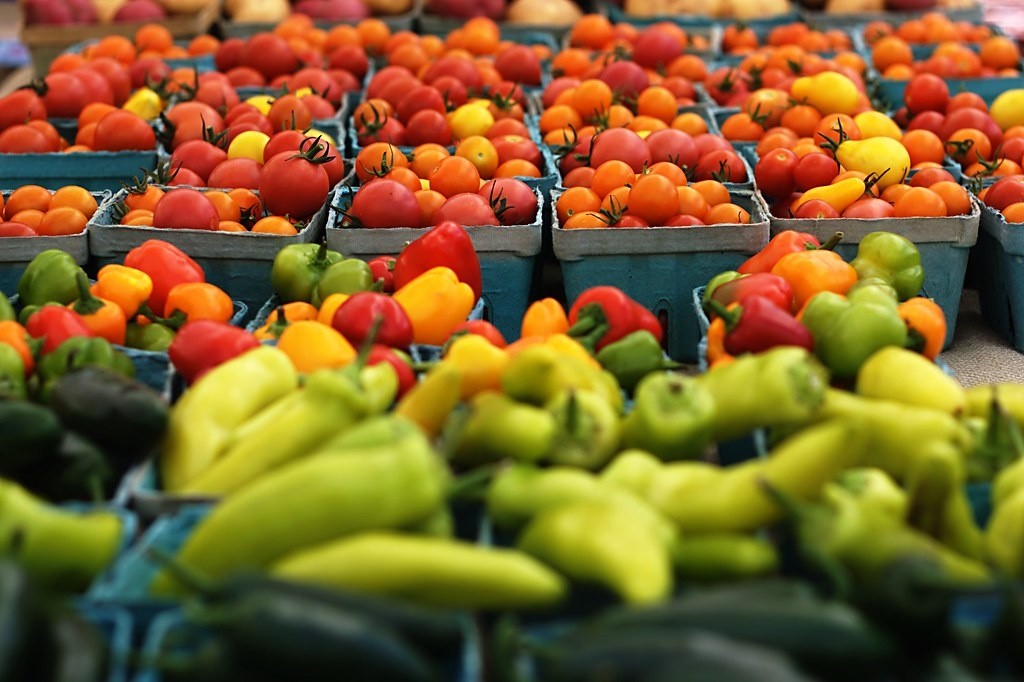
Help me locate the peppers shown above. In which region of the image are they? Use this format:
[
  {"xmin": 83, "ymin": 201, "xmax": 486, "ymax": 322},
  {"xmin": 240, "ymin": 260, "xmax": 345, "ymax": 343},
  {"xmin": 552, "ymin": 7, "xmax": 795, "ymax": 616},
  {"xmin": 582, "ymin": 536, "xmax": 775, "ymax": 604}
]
[{"xmin": 0, "ymin": 219, "xmax": 1023, "ymax": 682}]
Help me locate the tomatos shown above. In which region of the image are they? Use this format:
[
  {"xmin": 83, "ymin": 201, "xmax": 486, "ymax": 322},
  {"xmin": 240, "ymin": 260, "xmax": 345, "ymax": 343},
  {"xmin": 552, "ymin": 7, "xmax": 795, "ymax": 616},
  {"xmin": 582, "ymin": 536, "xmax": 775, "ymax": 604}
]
[{"xmin": 0, "ymin": 12, "xmax": 1023, "ymax": 237}]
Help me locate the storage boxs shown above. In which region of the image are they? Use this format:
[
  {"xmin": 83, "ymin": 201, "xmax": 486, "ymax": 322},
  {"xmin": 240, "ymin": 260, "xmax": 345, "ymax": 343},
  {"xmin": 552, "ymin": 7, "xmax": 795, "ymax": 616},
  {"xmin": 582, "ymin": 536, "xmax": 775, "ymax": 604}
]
[{"xmin": 0, "ymin": 0, "xmax": 1024, "ymax": 682}]
